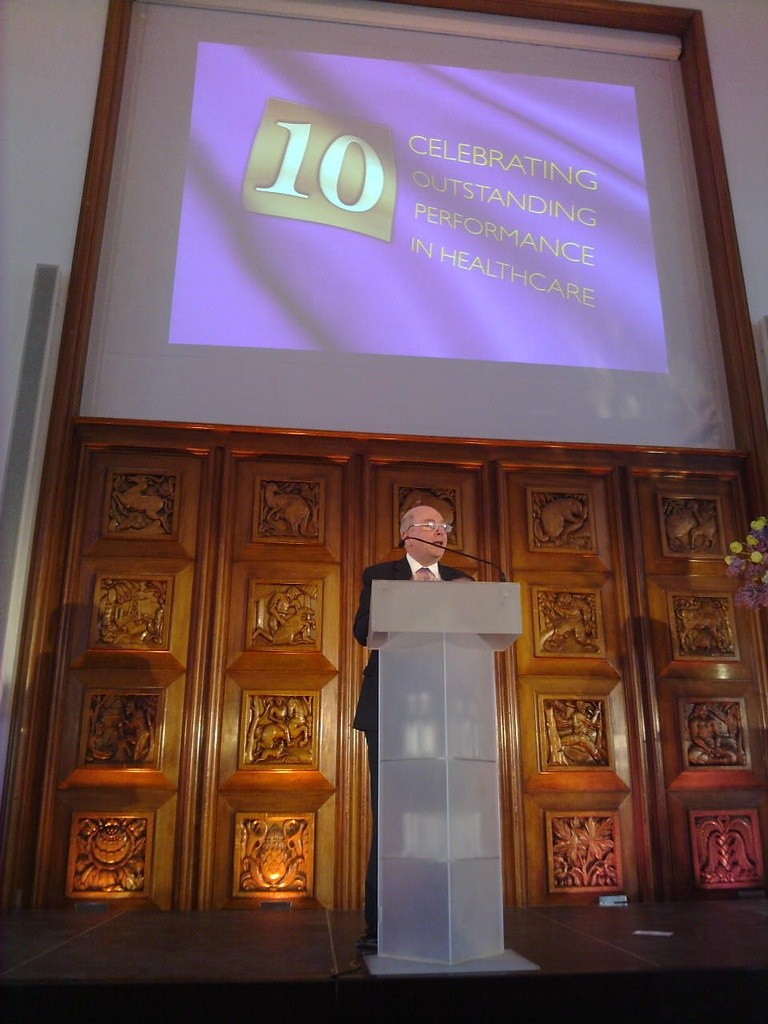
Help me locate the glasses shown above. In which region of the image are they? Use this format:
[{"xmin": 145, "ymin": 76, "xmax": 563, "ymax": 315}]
[{"xmin": 408, "ymin": 521, "xmax": 453, "ymax": 533}]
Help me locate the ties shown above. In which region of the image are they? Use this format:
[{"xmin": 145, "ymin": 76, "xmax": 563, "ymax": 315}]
[{"xmin": 416, "ymin": 568, "xmax": 430, "ymax": 581}]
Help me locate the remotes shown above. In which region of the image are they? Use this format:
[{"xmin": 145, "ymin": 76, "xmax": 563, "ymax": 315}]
[{"xmin": 398, "ymin": 536, "xmax": 507, "ymax": 582}]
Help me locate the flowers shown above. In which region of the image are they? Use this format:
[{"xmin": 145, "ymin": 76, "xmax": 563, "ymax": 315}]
[{"xmin": 725, "ymin": 516, "xmax": 767, "ymax": 610}]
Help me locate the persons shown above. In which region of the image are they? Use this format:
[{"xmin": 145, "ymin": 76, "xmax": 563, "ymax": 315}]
[{"xmin": 343, "ymin": 506, "xmax": 477, "ymax": 951}]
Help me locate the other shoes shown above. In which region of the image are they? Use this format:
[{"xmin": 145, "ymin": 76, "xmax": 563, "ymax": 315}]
[{"xmin": 356, "ymin": 924, "xmax": 377, "ymax": 949}]
